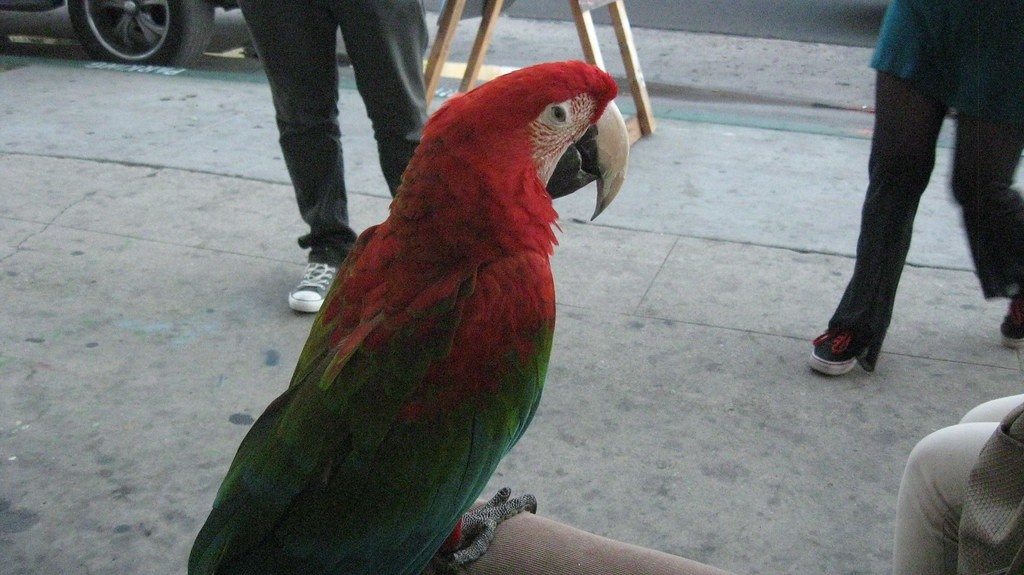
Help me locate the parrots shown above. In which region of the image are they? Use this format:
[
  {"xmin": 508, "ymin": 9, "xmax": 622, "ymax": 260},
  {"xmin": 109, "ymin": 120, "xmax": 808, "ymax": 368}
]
[{"xmin": 187, "ymin": 60, "xmax": 630, "ymax": 575}]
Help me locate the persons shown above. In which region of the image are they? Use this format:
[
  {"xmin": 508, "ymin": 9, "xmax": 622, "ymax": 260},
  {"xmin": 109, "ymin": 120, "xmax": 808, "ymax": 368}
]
[
  {"xmin": 809, "ymin": 0, "xmax": 1024, "ymax": 375},
  {"xmin": 237, "ymin": 1, "xmax": 430, "ymax": 313},
  {"xmin": 891, "ymin": 392, "xmax": 1024, "ymax": 574}
]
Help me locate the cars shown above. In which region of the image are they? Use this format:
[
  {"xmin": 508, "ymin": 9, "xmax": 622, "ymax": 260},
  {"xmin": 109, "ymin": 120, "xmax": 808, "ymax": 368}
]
[{"xmin": 0, "ymin": 1, "xmax": 242, "ymax": 69}]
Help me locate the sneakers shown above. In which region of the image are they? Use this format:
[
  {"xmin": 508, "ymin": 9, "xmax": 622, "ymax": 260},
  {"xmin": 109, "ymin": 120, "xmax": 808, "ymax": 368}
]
[
  {"xmin": 1000, "ymin": 295, "xmax": 1024, "ymax": 347},
  {"xmin": 811, "ymin": 329, "xmax": 867, "ymax": 377},
  {"xmin": 288, "ymin": 261, "xmax": 338, "ymax": 313}
]
